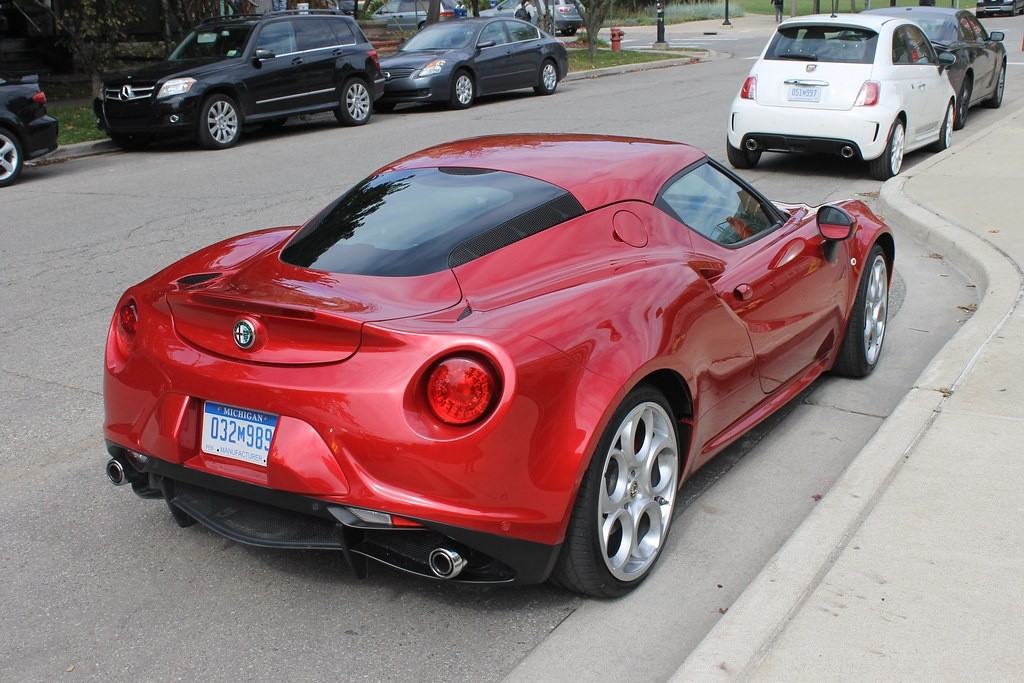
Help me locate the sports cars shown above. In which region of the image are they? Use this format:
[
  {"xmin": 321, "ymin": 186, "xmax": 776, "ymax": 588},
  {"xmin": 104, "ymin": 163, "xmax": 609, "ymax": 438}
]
[{"xmin": 101, "ymin": 133, "xmax": 898, "ymax": 598}]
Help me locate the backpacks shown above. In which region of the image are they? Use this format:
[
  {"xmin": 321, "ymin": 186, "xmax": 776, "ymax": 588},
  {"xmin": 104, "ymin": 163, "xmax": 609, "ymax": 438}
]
[{"xmin": 515, "ymin": 3, "xmax": 529, "ymax": 20}]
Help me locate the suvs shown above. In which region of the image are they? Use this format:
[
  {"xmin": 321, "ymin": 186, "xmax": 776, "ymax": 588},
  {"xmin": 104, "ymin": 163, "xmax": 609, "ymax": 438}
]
[{"xmin": 92, "ymin": 9, "xmax": 386, "ymax": 150}]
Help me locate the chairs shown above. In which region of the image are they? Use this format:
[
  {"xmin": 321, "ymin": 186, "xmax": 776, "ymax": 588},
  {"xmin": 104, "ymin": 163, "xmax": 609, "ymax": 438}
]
[
  {"xmin": 206, "ymin": 35, "xmax": 238, "ymax": 58},
  {"xmin": 863, "ymin": 29, "xmax": 904, "ymax": 63},
  {"xmin": 451, "ymin": 31, "xmax": 466, "ymax": 48},
  {"xmin": 802, "ymin": 31, "xmax": 826, "ymax": 50}
]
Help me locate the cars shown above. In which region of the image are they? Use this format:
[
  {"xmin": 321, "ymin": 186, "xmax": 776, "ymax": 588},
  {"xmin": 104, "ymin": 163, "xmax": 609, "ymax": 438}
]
[
  {"xmin": 838, "ymin": 6, "xmax": 1007, "ymax": 133},
  {"xmin": 975, "ymin": 0, "xmax": 1024, "ymax": 16},
  {"xmin": 479, "ymin": 0, "xmax": 586, "ymax": 35},
  {"xmin": 728, "ymin": 13, "xmax": 957, "ymax": 182},
  {"xmin": 373, "ymin": 16, "xmax": 570, "ymax": 111},
  {"xmin": 369, "ymin": 0, "xmax": 467, "ymax": 31},
  {"xmin": 1, "ymin": 74, "xmax": 61, "ymax": 187}
]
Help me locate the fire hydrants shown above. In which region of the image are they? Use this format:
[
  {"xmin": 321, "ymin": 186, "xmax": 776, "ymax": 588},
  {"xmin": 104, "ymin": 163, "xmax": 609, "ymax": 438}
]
[{"xmin": 609, "ymin": 26, "xmax": 625, "ymax": 51}]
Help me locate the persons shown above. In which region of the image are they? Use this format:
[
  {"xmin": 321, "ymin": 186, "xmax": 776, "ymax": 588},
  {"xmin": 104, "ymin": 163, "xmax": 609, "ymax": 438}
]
[
  {"xmin": 454, "ymin": 0, "xmax": 467, "ymax": 19},
  {"xmin": 514, "ymin": 0, "xmax": 535, "ymax": 23}
]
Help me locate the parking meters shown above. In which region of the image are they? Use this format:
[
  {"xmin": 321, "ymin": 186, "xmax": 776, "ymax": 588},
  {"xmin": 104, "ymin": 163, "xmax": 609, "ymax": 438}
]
[{"xmin": 454, "ymin": 4, "xmax": 467, "ymax": 18}]
